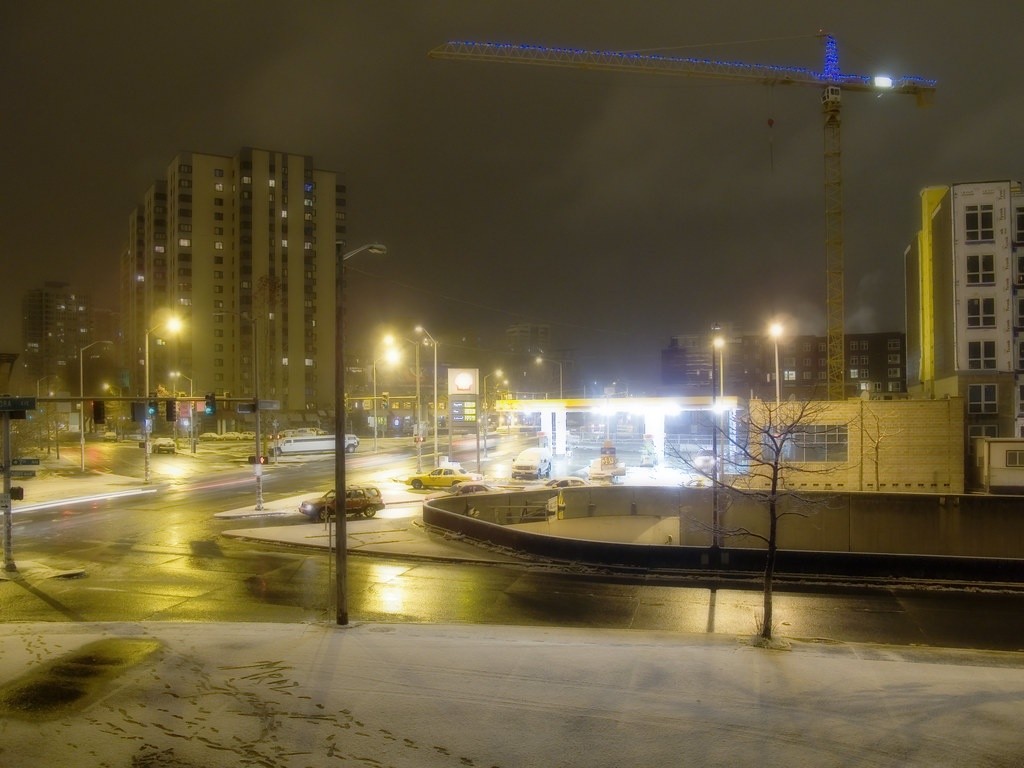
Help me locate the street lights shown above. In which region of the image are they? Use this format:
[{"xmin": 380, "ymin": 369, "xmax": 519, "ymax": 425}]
[
  {"xmin": 414, "ymin": 326, "xmax": 439, "ymax": 470},
  {"xmin": 81, "ymin": 339, "xmax": 116, "ymax": 473},
  {"xmin": 767, "ymin": 322, "xmax": 782, "ymax": 434},
  {"xmin": 711, "ymin": 337, "xmax": 725, "ymax": 486},
  {"xmin": 583, "ymin": 381, "xmax": 596, "ymax": 399},
  {"xmin": 372, "ymin": 353, "xmax": 399, "ymax": 453},
  {"xmin": 36, "ymin": 374, "xmax": 60, "ymax": 449},
  {"xmin": 612, "ymin": 381, "xmax": 629, "ymax": 398},
  {"xmin": 144, "ymin": 316, "xmax": 182, "ymax": 484},
  {"xmin": 482, "ymin": 369, "xmax": 502, "ymax": 458},
  {"xmin": 383, "ymin": 335, "xmax": 423, "ymax": 471},
  {"xmin": 335, "ymin": 237, "xmax": 388, "ymax": 626},
  {"xmin": 212, "ymin": 310, "xmax": 264, "ymax": 513},
  {"xmin": 175, "ymin": 372, "xmax": 194, "ymax": 452},
  {"xmin": 535, "ymin": 356, "xmax": 562, "ymax": 400},
  {"xmin": 503, "ymin": 380, "xmax": 509, "ymax": 401}
]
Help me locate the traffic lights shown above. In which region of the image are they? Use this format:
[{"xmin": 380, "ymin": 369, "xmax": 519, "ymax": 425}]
[
  {"xmin": 148, "ymin": 400, "xmax": 158, "ymax": 416},
  {"xmin": 204, "ymin": 394, "xmax": 216, "ymax": 415},
  {"xmin": 382, "ymin": 392, "xmax": 390, "ymax": 409}
]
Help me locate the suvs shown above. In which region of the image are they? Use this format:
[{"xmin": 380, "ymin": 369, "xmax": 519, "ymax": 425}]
[
  {"xmin": 695, "ymin": 448, "xmax": 721, "ymax": 475},
  {"xmin": 512, "ymin": 447, "xmax": 553, "ymax": 479},
  {"xmin": 298, "ymin": 483, "xmax": 386, "ymax": 522}
]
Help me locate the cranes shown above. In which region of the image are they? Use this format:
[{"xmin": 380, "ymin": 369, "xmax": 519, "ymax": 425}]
[{"xmin": 424, "ymin": 30, "xmax": 938, "ymax": 404}]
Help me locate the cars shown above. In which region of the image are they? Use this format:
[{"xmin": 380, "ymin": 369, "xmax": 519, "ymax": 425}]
[
  {"xmin": 523, "ymin": 476, "xmax": 601, "ymax": 492},
  {"xmin": 405, "ymin": 466, "xmax": 484, "ymax": 490},
  {"xmin": 153, "ymin": 438, "xmax": 176, "ymax": 455},
  {"xmin": 268, "ymin": 434, "xmax": 359, "ymax": 458},
  {"xmin": 424, "ymin": 481, "xmax": 505, "ymax": 503}
]
